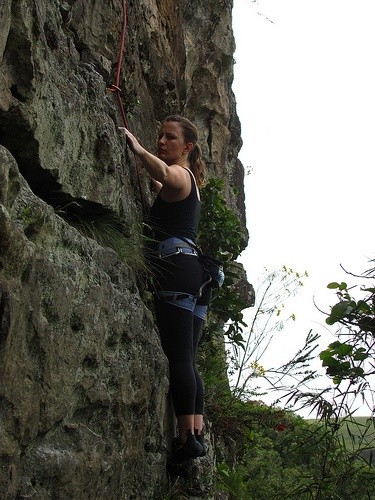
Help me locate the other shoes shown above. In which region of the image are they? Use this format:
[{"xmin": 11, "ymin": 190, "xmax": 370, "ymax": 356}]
[{"xmin": 173, "ymin": 428, "xmax": 206, "ymax": 460}]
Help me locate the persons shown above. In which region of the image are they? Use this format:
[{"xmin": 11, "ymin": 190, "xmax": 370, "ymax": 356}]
[{"xmin": 117, "ymin": 114, "xmax": 213, "ymax": 464}]
